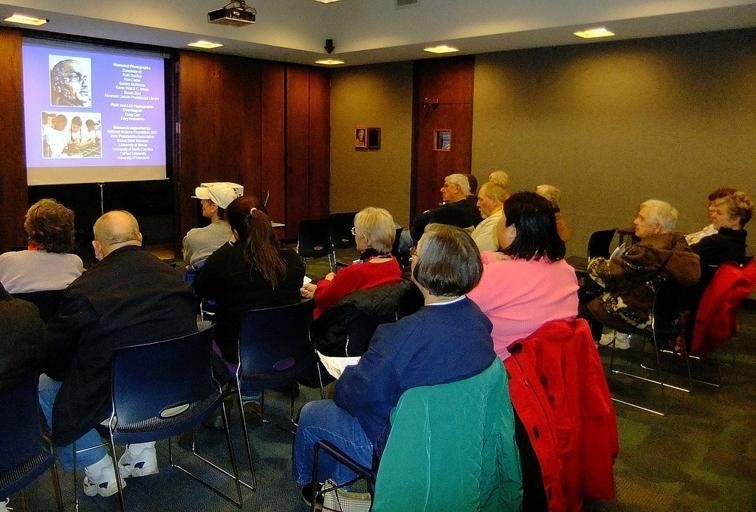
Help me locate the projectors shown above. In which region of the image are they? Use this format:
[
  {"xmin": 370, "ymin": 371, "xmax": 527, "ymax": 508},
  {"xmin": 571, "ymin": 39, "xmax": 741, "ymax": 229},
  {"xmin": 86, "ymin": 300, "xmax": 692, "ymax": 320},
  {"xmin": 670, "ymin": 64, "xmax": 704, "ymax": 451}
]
[{"xmin": 207, "ymin": 9, "xmax": 255, "ymax": 28}]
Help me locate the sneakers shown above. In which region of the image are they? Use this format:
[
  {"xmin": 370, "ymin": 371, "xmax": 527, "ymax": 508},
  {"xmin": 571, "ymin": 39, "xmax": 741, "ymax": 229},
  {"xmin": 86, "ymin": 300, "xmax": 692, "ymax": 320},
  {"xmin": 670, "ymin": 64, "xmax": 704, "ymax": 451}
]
[
  {"xmin": 83, "ymin": 456, "xmax": 125, "ymax": 497},
  {"xmin": 598, "ymin": 331, "xmax": 629, "ymax": 350},
  {"xmin": 117, "ymin": 449, "xmax": 159, "ymax": 480},
  {"xmin": 301, "ymin": 483, "xmax": 323, "ymax": 510}
]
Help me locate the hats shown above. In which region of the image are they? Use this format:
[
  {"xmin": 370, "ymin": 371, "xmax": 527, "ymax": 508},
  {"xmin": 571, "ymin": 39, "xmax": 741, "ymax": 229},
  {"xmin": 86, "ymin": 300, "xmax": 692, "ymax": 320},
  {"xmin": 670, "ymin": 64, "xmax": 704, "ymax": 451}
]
[{"xmin": 194, "ymin": 185, "xmax": 237, "ymax": 209}]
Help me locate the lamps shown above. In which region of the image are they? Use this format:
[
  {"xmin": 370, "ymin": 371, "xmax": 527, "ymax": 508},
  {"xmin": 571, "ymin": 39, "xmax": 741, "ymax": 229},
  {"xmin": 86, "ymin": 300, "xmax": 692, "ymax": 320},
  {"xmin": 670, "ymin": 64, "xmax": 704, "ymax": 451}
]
[{"xmin": 207, "ymin": 0, "xmax": 257, "ymax": 26}]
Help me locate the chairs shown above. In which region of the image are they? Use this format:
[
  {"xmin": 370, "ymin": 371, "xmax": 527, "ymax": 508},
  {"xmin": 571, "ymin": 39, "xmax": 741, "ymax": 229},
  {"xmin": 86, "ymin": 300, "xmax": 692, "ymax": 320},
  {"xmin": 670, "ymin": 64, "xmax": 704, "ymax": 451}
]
[
  {"xmin": 319, "ymin": 272, "xmax": 427, "ymax": 353},
  {"xmin": 606, "ymin": 285, "xmax": 697, "ymax": 417},
  {"xmin": 560, "ymin": 226, "xmax": 615, "ymax": 279},
  {"xmin": 313, "ymin": 367, "xmax": 505, "ymax": 512},
  {"xmin": 506, "ymin": 313, "xmax": 620, "ymax": 509},
  {"xmin": 298, "ymin": 212, "xmax": 401, "ymax": 263},
  {"xmin": 0, "ymin": 358, "xmax": 65, "ymax": 512},
  {"xmin": 74, "ymin": 324, "xmax": 244, "ymax": 511},
  {"xmin": 193, "ymin": 298, "xmax": 325, "ymax": 493},
  {"xmin": 702, "ymin": 255, "xmax": 754, "ymax": 392}
]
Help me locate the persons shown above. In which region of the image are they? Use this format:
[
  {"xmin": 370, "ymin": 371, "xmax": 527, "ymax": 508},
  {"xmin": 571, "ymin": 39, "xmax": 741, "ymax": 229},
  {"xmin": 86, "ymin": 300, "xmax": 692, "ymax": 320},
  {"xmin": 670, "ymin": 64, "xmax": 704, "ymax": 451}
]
[
  {"xmin": 42, "ymin": 111, "xmax": 102, "ymax": 159},
  {"xmin": 470, "ymin": 170, "xmax": 511, "ymax": 249},
  {"xmin": 182, "ymin": 183, "xmax": 237, "ymax": 270},
  {"xmin": 0, "ymin": 199, "xmax": 83, "ymax": 292},
  {"xmin": 398, "ymin": 173, "xmax": 484, "ymax": 252},
  {"xmin": 300, "ymin": 206, "xmax": 401, "ymax": 319},
  {"xmin": 51, "ymin": 58, "xmax": 91, "ymax": 106},
  {"xmin": 684, "ymin": 186, "xmax": 753, "ymax": 268},
  {"xmin": 537, "ymin": 185, "xmax": 570, "ymax": 243},
  {"xmin": 356, "ymin": 129, "xmax": 365, "ymax": 146},
  {"xmin": 41, "ymin": 211, "xmax": 184, "ymax": 497},
  {"xmin": 293, "ymin": 223, "xmax": 497, "ymax": 510},
  {"xmin": 466, "ymin": 191, "xmax": 580, "ymax": 358},
  {"xmin": 585, "ymin": 200, "xmax": 700, "ymax": 350},
  {"xmin": 201, "ymin": 196, "xmax": 306, "ymax": 421}
]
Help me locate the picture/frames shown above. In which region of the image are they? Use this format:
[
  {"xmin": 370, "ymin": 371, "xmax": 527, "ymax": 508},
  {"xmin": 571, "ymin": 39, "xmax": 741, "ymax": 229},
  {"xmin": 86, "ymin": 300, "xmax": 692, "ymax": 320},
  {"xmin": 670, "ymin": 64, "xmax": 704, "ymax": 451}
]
[
  {"xmin": 354, "ymin": 128, "xmax": 381, "ymax": 150},
  {"xmin": 433, "ymin": 129, "xmax": 452, "ymax": 152}
]
